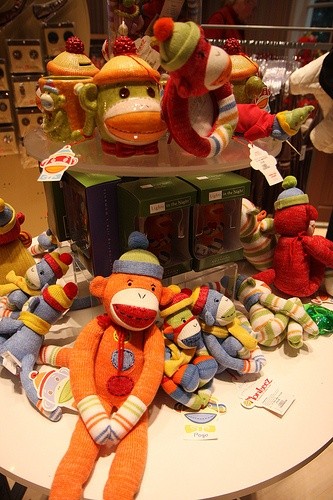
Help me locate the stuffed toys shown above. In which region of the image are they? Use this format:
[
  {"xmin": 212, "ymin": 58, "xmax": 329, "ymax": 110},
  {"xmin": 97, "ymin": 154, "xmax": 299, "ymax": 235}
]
[
  {"xmin": 252, "ymin": 175, "xmax": 333, "ymax": 297},
  {"xmin": 49, "ymin": 231, "xmax": 173, "ymax": 500},
  {"xmin": 197, "ymin": 203, "xmax": 225, "ymax": 255},
  {"xmin": 221, "ymin": 274, "xmax": 319, "ymax": 349},
  {"xmin": 0, "ymin": 197, "xmax": 78, "ymax": 422},
  {"xmin": 181, "ymin": 286, "xmax": 266, "ymax": 376},
  {"xmin": 239, "ymin": 198, "xmax": 277, "ymax": 272},
  {"xmin": 154, "ymin": 18, "xmax": 239, "ymax": 160},
  {"xmin": 145, "ymin": 214, "xmax": 176, "ymax": 262},
  {"xmin": 157, "ymin": 285, "xmax": 217, "ymax": 410},
  {"xmin": 234, "ymin": 105, "xmax": 315, "ymax": 142}
]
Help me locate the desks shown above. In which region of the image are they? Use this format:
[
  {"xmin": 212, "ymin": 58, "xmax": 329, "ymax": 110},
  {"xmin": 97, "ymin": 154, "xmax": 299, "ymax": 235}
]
[
  {"xmin": 24, "ymin": 125, "xmax": 282, "ymax": 179},
  {"xmin": 0, "ymin": 295, "xmax": 333, "ymax": 500}
]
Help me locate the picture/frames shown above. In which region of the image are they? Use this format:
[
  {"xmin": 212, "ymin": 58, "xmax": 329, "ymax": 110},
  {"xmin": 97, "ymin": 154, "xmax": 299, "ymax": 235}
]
[{"xmin": 197, "ymin": 0, "xmax": 297, "ymax": 61}]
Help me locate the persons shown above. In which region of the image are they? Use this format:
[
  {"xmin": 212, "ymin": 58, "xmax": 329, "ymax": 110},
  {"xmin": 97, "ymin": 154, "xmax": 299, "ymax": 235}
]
[{"xmin": 204, "ymin": 0, "xmax": 257, "ymax": 55}]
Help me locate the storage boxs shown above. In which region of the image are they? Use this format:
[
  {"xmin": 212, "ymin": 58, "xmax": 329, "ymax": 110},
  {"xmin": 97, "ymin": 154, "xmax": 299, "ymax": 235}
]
[
  {"xmin": 61, "ymin": 170, "xmax": 122, "ymax": 279},
  {"xmin": 167, "ymin": 262, "xmax": 238, "ymax": 303},
  {"xmin": 10, "ymin": 74, "xmax": 43, "ymax": 110},
  {"xmin": 14, "ymin": 108, "xmax": 44, "ymax": 141},
  {"xmin": 0, "ymin": 124, "xmax": 19, "ymax": 157},
  {"xmin": 0, "ymin": 57, "xmax": 10, "ymax": 94},
  {"xmin": 5, "ymin": 39, "xmax": 46, "ymax": 75},
  {"xmin": 42, "ymin": 21, "xmax": 76, "ymax": 59},
  {"xmin": 17, "ymin": 139, "xmax": 39, "ymax": 169},
  {"xmin": 0, "ymin": 94, "xmax": 15, "ymax": 126},
  {"xmin": 117, "ymin": 176, "xmax": 197, "ymax": 279},
  {"xmin": 55, "ymin": 241, "xmax": 103, "ymax": 314},
  {"xmin": 38, "ymin": 160, "xmax": 70, "ymax": 242},
  {"xmin": 175, "ymin": 171, "xmax": 252, "ymax": 273}
]
